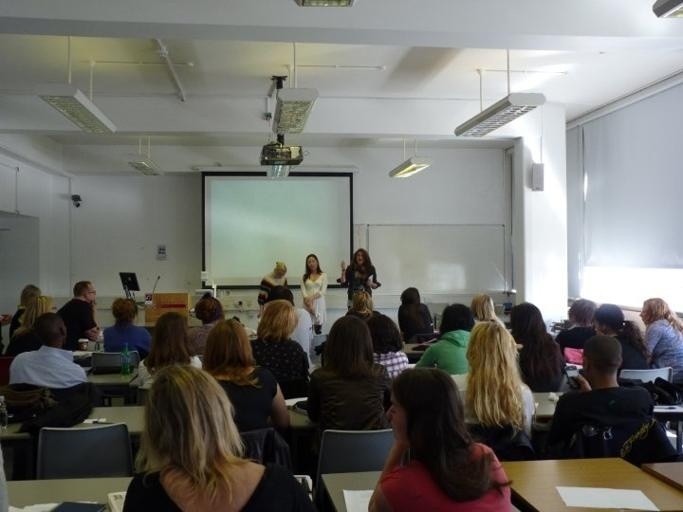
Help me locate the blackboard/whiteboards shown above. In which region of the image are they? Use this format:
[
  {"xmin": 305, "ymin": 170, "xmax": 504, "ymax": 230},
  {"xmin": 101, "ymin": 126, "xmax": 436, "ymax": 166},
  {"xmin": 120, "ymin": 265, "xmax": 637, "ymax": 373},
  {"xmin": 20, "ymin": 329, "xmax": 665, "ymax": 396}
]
[{"xmin": 367, "ymin": 223, "xmax": 507, "ymax": 295}]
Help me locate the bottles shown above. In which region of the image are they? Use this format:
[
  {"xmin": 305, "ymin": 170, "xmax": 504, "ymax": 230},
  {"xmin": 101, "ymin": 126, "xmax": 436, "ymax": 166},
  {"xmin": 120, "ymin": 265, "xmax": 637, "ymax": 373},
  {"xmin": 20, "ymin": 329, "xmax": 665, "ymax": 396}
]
[
  {"xmin": 0, "ymin": 396, "xmax": 8, "ymax": 433},
  {"xmin": 118, "ymin": 342, "xmax": 131, "ymax": 381}
]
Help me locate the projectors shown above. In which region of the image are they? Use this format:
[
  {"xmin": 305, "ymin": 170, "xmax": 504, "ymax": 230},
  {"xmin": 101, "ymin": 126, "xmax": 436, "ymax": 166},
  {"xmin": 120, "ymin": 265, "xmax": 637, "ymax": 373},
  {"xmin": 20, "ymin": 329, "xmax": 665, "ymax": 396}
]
[{"xmin": 260, "ymin": 144, "xmax": 303, "ymax": 165}]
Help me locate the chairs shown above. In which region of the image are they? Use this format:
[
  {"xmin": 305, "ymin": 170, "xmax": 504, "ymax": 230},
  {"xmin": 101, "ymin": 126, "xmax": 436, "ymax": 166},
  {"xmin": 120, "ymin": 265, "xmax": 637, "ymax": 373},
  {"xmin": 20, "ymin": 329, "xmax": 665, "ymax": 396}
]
[{"xmin": 1, "ymin": 343, "xmax": 683, "ymax": 512}]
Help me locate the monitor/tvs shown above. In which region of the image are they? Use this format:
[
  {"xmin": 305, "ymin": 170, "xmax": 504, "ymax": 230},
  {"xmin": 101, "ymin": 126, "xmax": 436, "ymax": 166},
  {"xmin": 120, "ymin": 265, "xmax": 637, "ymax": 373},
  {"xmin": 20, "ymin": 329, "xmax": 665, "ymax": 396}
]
[{"xmin": 119, "ymin": 271, "xmax": 140, "ymax": 291}]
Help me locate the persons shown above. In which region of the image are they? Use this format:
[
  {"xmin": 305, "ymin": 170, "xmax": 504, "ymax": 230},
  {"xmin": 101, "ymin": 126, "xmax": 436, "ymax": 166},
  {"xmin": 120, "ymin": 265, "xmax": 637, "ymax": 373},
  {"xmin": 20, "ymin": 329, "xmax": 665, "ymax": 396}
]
[
  {"xmin": 368, "ymin": 368, "xmax": 513, "ymax": 511},
  {"xmin": 0, "ymin": 282, "xmax": 683, "ymax": 460},
  {"xmin": 122, "ymin": 360, "xmax": 315, "ymax": 511},
  {"xmin": 257, "ymin": 261, "xmax": 289, "ymax": 318},
  {"xmin": 340, "ymin": 248, "xmax": 381, "ymax": 309},
  {"xmin": 299, "ymin": 253, "xmax": 328, "ymax": 336}
]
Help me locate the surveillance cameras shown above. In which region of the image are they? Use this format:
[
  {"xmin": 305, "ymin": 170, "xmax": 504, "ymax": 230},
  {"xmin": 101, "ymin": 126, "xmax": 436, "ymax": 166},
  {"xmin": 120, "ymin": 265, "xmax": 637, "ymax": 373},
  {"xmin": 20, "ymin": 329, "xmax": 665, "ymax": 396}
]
[{"xmin": 70, "ymin": 193, "xmax": 82, "ymax": 207}]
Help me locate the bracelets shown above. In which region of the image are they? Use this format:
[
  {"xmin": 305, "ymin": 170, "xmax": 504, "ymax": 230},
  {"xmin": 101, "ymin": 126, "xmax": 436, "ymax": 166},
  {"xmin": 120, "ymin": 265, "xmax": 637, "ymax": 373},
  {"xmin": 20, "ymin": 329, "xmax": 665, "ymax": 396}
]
[{"xmin": 342, "ymin": 268, "xmax": 346, "ymax": 271}]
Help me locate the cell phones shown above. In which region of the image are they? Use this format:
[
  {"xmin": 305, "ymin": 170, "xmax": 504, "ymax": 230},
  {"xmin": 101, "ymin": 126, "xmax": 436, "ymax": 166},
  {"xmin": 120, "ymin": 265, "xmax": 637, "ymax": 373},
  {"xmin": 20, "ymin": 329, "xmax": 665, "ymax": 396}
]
[{"xmin": 564, "ymin": 365, "xmax": 581, "ymax": 389}]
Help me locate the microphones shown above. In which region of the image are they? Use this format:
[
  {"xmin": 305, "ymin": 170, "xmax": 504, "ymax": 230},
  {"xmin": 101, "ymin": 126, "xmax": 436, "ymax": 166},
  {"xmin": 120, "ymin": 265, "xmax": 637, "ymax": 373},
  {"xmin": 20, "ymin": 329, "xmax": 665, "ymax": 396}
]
[{"xmin": 151, "ymin": 275, "xmax": 161, "ymax": 293}]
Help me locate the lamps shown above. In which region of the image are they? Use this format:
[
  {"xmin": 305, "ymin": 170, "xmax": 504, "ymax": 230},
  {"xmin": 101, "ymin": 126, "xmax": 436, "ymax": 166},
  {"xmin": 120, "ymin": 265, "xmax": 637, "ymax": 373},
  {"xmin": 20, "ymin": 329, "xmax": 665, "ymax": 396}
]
[
  {"xmin": 653, "ymin": 1, "xmax": 683, "ymax": 20},
  {"xmin": 35, "ymin": 36, "xmax": 116, "ymax": 135},
  {"xmin": 128, "ymin": 137, "xmax": 165, "ymax": 176},
  {"xmin": 390, "ymin": 139, "xmax": 432, "ymax": 178},
  {"xmin": 273, "ymin": 42, "xmax": 318, "ymax": 135},
  {"xmin": 454, "ymin": 48, "xmax": 545, "ymax": 137}
]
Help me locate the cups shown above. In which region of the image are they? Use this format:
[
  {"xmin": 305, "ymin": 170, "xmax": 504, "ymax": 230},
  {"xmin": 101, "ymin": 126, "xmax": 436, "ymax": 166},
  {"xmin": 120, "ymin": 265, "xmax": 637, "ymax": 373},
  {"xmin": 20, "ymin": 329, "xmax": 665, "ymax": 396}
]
[{"xmin": 78, "ymin": 338, "xmax": 89, "ymax": 351}]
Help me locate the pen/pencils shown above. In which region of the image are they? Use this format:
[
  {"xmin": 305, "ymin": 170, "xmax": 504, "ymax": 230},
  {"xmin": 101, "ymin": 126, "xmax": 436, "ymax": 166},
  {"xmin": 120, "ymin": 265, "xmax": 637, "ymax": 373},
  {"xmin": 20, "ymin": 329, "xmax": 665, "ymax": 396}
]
[
  {"xmin": 93, "ymin": 421, "xmax": 114, "ymax": 425},
  {"xmin": 654, "ymin": 406, "xmax": 676, "ymax": 409},
  {"xmin": 129, "ymin": 374, "xmax": 139, "ymax": 383}
]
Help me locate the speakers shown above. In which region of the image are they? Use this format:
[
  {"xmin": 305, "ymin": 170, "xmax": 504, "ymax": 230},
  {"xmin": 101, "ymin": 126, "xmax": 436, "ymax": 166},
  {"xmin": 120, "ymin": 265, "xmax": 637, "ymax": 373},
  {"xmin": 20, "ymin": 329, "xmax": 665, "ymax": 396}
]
[{"xmin": 531, "ymin": 164, "xmax": 544, "ymax": 190}]
